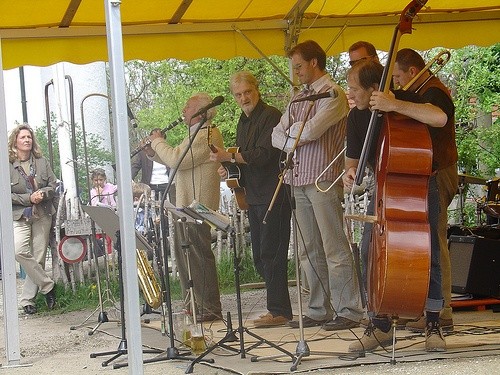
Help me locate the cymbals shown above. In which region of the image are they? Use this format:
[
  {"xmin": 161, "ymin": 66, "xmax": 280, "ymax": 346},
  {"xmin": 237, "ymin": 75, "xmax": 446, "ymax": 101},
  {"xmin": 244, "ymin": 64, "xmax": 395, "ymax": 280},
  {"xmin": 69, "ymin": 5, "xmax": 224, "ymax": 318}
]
[
  {"xmin": 344, "ymin": 215, "xmax": 378, "ymax": 223},
  {"xmin": 458, "ymin": 174, "xmax": 489, "ymax": 185},
  {"xmin": 113, "ymin": 183, "xmax": 151, "ymax": 203}
]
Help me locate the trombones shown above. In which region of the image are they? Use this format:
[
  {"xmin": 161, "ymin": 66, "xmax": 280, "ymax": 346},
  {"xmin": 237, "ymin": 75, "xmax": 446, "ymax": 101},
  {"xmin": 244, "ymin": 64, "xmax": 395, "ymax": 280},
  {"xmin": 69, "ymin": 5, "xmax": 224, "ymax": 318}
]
[{"xmin": 314, "ymin": 50, "xmax": 452, "ymax": 193}]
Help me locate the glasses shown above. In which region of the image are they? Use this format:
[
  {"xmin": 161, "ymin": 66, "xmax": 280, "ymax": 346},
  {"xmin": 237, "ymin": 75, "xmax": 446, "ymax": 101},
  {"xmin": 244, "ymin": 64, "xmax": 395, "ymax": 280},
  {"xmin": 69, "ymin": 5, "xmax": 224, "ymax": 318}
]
[
  {"xmin": 349, "ymin": 54, "xmax": 373, "ymax": 67},
  {"xmin": 292, "ymin": 60, "xmax": 306, "ymax": 71}
]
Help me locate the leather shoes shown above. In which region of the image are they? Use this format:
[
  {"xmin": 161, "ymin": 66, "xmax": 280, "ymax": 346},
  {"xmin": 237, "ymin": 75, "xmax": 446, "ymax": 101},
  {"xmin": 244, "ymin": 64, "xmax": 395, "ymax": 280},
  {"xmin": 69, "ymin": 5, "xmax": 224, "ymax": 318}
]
[
  {"xmin": 23, "ymin": 305, "xmax": 37, "ymax": 314},
  {"xmin": 44, "ymin": 281, "xmax": 57, "ymax": 310}
]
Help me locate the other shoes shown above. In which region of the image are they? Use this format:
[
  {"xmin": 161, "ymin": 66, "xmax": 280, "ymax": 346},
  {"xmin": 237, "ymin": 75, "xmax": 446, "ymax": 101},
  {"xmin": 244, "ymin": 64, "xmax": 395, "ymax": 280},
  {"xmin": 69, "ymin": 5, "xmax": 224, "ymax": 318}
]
[
  {"xmin": 288, "ymin": 315, "xmax": 333, "ymax": 328},
  {"xmin": 191, "ymin": 310, "xmax": 224, "ymax": 323},
  {"xmin": 178, "ymin": 305, "xmax": 200, "ymax": 316},
  {"xmin": 321, "ymin": 316, "xmax": 361, "ymax": 331}
]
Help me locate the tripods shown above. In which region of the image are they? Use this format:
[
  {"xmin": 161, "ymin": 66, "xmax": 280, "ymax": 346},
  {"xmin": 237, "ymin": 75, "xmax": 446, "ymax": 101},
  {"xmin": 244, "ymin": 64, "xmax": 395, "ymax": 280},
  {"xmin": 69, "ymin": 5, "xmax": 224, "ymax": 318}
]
[{"xmin": 70, "ymin": 94, "xmax": 365, "ymax": 373}]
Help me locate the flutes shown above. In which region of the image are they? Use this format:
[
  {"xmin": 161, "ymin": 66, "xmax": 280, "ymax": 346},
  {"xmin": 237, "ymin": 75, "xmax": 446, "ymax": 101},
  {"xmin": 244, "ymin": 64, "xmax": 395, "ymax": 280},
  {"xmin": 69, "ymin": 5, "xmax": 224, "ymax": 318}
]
[{"xmin": 111, "ymin": 116, "xmax": 185, "ymax": 171}]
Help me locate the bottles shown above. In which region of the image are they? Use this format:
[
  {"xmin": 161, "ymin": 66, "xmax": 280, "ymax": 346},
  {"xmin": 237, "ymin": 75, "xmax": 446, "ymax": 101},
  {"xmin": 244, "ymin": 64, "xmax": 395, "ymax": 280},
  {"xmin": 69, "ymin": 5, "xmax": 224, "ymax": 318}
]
[{"xmin": 182, "ymin": 308, "xmax": 194, "ymax": 350}]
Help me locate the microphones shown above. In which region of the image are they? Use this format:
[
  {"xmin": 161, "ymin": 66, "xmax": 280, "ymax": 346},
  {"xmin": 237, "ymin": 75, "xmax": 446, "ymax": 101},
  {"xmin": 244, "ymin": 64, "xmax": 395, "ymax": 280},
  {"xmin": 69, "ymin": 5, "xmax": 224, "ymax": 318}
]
[
  {"xmin": 293, "ymin": 89, "xmax": 338, "ymax": 103},
  {"xmin": 191, "ymin": 96, "xmax": 224, "ymax": 118},
  {"xmin": 126, "ymin": 104, "xmax": 138, "ymax": 128}
]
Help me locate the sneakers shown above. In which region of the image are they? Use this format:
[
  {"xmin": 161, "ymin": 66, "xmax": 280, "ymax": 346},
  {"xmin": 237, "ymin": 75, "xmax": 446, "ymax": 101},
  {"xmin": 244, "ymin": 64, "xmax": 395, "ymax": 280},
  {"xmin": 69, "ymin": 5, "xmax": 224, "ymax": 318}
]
[
  {"xmin": 422, "ymin": 321, "xmax": 447, "ymax": 351},
  {"xmin": 407, "ymin": 313, "xmax": 454, "ymax": 332},
  {"xmin": 348, "ymin": 323, "xmax": 397, "ymax": 351},
  {"xmin": 254, "ymin": 312, "xmax": 292, "ymax": 325},
  {"xmin": 392, "ymin": 312, "xmax": 424, "ymax": 330}
]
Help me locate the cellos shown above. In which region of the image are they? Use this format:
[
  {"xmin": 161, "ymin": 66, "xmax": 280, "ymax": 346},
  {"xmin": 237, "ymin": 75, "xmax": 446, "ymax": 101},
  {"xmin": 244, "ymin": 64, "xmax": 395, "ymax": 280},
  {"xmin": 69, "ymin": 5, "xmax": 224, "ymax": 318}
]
[{"xmin": 343, "ymin": 0, "xmax": 435, "ymax": 366}]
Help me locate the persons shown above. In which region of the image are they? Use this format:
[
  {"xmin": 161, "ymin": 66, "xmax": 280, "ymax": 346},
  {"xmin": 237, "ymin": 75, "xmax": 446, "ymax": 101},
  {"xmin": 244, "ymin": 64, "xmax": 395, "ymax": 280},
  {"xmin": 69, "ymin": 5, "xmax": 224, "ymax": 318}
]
[
  {"xmin": 270, "ymin": 41, "xmax": 363, "ymax": 330},
  {"xmin": 344, "ymin": 41, "xmax": 460, "ymax": 355},
  {"xmin": 217, "ymin": 73, "xmax": 296, "ymax": 327},
  {"xmin": 90, "ymin": 168, "xmax": 117, "ymax": 212},
  {"xmin": 132, "ymin": 182, "xmax": 161, "ymax": 237},
  {"xmin": 141, "ymin": 93, "xmax": 227, "ymax": 322},
  {"xmin": 8, "ymin": 123, "xmax": 58, "ymax": 313}
]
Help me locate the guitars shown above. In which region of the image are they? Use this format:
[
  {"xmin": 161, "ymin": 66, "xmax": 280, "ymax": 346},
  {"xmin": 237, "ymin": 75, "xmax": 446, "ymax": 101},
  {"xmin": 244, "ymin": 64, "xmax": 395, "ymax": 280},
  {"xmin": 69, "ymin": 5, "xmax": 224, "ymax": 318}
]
[{"xmin": 207, "ymin": 126, "xmax": 249, "ymax": 210}]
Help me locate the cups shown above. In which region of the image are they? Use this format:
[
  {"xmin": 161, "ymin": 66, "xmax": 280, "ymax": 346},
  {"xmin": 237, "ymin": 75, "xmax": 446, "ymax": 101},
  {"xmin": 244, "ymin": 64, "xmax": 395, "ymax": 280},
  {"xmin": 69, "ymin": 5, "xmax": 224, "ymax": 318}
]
[{"xmin": 189, "ymin": 324, "xmax": 206, "ymax": 355}]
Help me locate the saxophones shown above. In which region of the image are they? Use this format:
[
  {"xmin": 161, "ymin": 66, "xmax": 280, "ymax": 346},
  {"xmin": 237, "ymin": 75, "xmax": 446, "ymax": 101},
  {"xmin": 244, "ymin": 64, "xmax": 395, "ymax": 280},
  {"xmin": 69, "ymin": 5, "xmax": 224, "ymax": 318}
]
[{"xmin": 137, "ymin": 249, "xmax": 162, "ymax": 309}]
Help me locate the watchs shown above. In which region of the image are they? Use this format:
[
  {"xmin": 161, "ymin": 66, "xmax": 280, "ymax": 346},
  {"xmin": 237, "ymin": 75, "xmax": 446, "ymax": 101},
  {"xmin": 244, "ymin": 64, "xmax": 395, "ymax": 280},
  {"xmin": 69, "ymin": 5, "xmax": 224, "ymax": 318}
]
[{"xmin": 231, "ymin": 153, "xmax": 236, "ymax": 164}]
